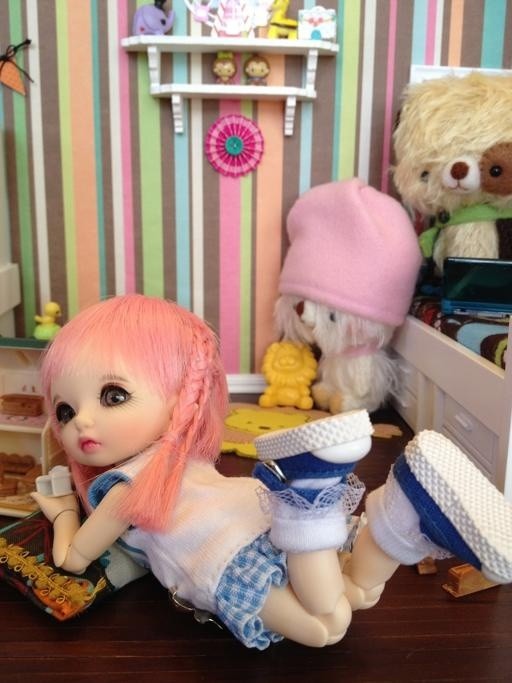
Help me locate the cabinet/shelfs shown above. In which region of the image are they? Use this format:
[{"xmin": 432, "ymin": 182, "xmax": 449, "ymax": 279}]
[{"xmin": 121, "ymin": 35, "xmax": 341, "ymax": 136}]
